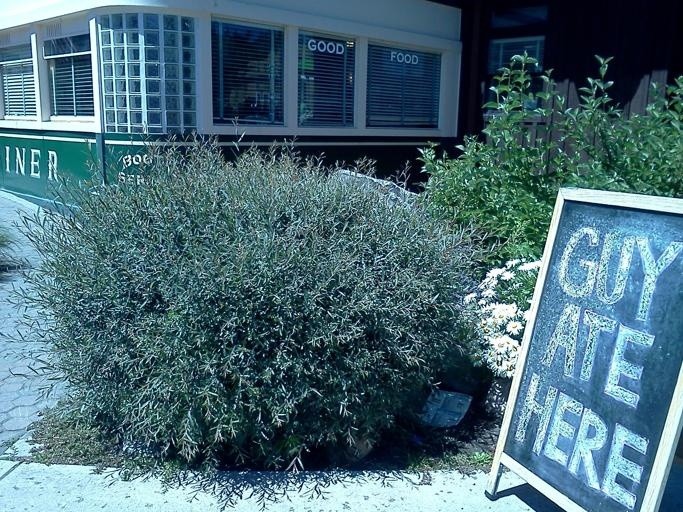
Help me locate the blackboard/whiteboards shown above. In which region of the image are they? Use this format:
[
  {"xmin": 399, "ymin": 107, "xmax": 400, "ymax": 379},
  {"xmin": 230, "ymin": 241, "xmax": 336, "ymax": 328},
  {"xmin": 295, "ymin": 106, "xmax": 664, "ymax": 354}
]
[{"xmin": 500, "ymin": 189, "xmax": 683, "ymax": 512}]
